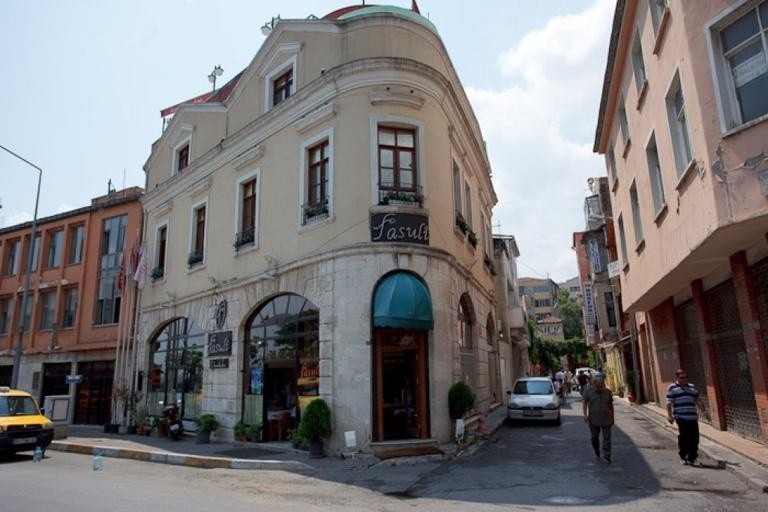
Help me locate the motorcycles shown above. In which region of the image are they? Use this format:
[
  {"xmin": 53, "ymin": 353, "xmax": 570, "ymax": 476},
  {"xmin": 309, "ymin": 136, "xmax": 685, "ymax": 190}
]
[{"xmin": 159, "ymin": 398, "xmax": 188, "ymax": 441}]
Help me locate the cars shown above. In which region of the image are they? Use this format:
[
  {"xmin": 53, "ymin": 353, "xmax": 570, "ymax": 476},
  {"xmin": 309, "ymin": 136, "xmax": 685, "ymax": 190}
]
[
  {"xmin": 505, "ymin": 376, "xmax": 562, "ymax": 426},
  {"xmin": 296, "ymin": 376, "xmax": 321, "ymax": 414},
  {"xmin": 571, "ymin": 366, "xmax": 597, "ymax": 391},
  {"xmin": 183, "ymin": 387, "xmax": 202, "ymax": 413}
]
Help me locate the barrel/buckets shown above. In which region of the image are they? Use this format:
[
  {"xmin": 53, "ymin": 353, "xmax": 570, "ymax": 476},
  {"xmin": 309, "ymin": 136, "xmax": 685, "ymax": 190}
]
[
  {"xmin": 33, "ymin": 447, "xmax": 42, "ymax": 463},
  {"xmin": 93, "ymin": 452, "xmax": 103, "ymax": 470}
]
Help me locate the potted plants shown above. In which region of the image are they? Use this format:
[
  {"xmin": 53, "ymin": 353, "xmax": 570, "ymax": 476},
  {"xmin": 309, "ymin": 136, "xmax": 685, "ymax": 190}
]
[
  {"xmin": 233, "ymin": 422, "xmax": 250, "ymax": 442},
  {"xmin": 193, "ymin": 414, "xmax": 219, "ymax": 445},
  {"xmin": 109, "ymin": 378, "xmax": 169, "ymax": 438},
  {"xmin": 248, "ymin": 424, "xmax": 262, "ymax": 443},
  {"xmin": 294, "ymin": 398, "xmax": 332, "ymax": 456},
  {"xmin": 292, "ymin": 426, "xmax": 314, "ymax": 452}
]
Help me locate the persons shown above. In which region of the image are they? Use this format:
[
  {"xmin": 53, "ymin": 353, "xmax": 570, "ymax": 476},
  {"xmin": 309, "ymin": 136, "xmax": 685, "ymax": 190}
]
[
  {"xmin": 580, "ymin": 374, "xmax": 614, "ymax": 465},
  {"xmin": 543, "ymin": 365, "xmax": 606, "ymax": 406},
  {"xmin": 664, "ymin": 370, "xmax": 711, "ymax": 467}
]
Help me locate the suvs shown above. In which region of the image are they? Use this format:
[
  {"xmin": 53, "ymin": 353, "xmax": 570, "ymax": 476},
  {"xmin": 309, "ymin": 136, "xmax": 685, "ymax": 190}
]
[{"xmin": 1, "ymin": 387, "xmax": 55, "ymax": 457}]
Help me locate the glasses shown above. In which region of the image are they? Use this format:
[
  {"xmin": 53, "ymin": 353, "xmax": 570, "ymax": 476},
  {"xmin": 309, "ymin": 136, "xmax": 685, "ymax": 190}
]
[{"xmin": 680, "ymin": 376, "xmax": 688, "ymax": 379}]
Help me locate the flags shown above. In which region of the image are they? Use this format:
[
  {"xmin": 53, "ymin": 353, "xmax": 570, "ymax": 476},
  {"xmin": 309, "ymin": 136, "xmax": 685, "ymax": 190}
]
[{"xmin": 117, "ymin": 246, "xmax": 146, "ymax": 296}]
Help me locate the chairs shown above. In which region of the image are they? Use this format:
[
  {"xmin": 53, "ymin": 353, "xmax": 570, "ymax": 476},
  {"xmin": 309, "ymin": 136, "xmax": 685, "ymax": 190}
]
[{"xmin": 271, "ymin": 409, "xmax": 290, "ymax": 441}]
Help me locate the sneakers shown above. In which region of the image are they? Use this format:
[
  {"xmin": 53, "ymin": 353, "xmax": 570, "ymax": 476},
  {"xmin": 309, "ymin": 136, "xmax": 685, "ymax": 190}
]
[
  {"xmin": 593, "ymin": 449, "xmax": 612, "ymax": 464},
  {"xmin": 679, "ymin": 458, "xmax": 702, "ymax": 467}
]
